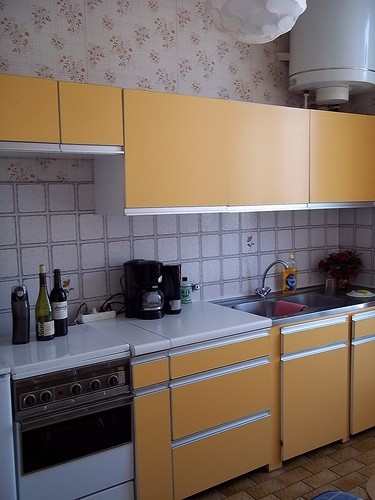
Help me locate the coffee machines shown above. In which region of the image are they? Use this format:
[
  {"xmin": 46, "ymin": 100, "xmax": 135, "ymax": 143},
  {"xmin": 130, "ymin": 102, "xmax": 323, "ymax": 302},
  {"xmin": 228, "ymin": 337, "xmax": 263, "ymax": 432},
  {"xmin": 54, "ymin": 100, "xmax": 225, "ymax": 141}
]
[{"xmin": 123, "ymin": 259, "xmax": 165, "ymax": 320}]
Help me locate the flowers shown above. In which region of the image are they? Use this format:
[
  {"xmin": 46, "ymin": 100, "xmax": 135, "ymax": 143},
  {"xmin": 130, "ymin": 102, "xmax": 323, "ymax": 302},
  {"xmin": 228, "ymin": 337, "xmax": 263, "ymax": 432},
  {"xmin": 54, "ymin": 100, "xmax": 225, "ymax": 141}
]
[{"xmin": 318, "ymin": 244, "xmax": 365, "ymax": 282}]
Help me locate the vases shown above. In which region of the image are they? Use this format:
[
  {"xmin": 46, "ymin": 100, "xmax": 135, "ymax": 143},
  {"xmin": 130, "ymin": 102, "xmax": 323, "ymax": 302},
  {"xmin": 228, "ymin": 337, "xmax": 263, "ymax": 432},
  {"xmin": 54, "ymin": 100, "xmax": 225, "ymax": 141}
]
[{"xmin": 337, "ymin": 279, "xmax": 348, "ymax": 288}]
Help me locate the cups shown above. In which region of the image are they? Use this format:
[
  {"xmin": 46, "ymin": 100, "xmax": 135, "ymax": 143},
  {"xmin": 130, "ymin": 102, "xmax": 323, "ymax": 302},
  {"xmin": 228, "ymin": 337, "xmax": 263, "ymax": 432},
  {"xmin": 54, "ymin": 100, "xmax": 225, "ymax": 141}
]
[{"xmin": 324, "ymin": 279, "xmax": 336, "ymax": 297}]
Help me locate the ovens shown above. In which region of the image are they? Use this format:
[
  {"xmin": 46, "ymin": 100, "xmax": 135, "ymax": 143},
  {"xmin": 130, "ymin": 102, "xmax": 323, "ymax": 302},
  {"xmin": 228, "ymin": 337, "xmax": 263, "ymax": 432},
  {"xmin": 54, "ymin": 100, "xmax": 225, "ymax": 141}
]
[{"xmin": 12, "ymin": 350, "xmax": 134, "ymax": 500}]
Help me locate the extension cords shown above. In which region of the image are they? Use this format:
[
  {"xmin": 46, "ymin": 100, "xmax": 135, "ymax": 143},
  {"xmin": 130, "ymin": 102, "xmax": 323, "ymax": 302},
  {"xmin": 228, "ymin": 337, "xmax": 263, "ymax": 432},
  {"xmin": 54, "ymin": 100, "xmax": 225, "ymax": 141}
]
[{"xmin": 78, "ymin": 310, "xmax": 117, "ymax": 323}]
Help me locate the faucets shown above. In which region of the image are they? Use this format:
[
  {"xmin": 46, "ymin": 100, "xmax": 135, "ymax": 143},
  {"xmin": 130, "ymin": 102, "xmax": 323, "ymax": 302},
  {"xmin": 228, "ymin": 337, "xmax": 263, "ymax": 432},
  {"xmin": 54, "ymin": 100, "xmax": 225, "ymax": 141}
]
[{"xmin": 254, "ymin": 259, "xmax": 289, "ymax": 299}]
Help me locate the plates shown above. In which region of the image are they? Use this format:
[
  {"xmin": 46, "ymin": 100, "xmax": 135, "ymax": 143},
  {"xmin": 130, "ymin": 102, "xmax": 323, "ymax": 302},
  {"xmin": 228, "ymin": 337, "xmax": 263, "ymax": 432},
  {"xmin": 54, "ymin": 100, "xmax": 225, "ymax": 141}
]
[{"xmin": 346, "ymin": 292, "xmax": 375, "ymax": 297}]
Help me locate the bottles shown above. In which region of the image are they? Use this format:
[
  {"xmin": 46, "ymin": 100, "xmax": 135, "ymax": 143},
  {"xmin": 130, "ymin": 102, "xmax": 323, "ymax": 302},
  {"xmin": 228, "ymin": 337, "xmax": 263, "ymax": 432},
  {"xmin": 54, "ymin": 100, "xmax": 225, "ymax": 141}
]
[
  {"xmin": 180, "ymin": 277, "xmax": 192, "ymax": 306},
  {"xmin": 35, "ymin": 265, "xmax": 55, "ymax": 341},
  {"xmin": 50, "ymin": 269, "xmax": 68, "ymax": 337}
]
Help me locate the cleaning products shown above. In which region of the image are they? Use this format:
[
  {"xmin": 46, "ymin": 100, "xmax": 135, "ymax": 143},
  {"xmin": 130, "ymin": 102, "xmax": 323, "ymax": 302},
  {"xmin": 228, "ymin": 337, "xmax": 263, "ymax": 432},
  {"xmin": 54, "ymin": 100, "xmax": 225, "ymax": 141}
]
[{"xmin": 282, "ymin": 249, "xmax": 297, "ymax": 296}]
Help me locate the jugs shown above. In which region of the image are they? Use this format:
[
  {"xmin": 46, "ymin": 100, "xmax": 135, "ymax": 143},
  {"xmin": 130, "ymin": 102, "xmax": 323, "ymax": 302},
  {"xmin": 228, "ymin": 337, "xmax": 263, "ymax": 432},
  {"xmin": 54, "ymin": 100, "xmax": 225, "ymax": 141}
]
[{"xmin": 142, "ymin": 287, "xmax": 164, "ymax": 310}]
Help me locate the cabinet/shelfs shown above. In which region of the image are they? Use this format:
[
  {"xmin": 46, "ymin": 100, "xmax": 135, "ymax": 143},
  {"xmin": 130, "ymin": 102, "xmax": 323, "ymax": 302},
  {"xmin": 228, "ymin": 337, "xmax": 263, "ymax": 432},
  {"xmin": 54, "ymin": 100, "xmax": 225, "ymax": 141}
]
[
  {"xmin": 122, "ymin": 87, "xmax": 229, "ymax": 216},
  {"xmin": 229, "ymin": 100, "xmax": 310, "ymax": 213},
  {"xmin": 352, "ymin": 309, "xmax": 375, "ymax": 435},
  {"xmin": 0, "ymin": 75, "xmax": 124, "ymax": 154},
  {"xmin": 277, "ymin": 311, "xmax": 350, "ymax": 469},
  {"xmin": 310, "ymin": 109, "xmax": 375, "ymax": 209},
  {"xmin": 131, "ymin": 331, "xmax": 274, "ymax": 500}
]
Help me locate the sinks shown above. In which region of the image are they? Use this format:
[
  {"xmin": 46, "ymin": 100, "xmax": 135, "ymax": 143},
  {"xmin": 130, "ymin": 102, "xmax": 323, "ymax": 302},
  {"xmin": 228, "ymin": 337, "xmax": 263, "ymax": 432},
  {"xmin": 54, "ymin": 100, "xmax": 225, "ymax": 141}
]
[
  {"xmin": 273, "ymin": 291, "xmax": 345, "ymax": 309},
  {"xmin": 224, "ymin": 297, "xmax": 313, "ymax": 322}
]
